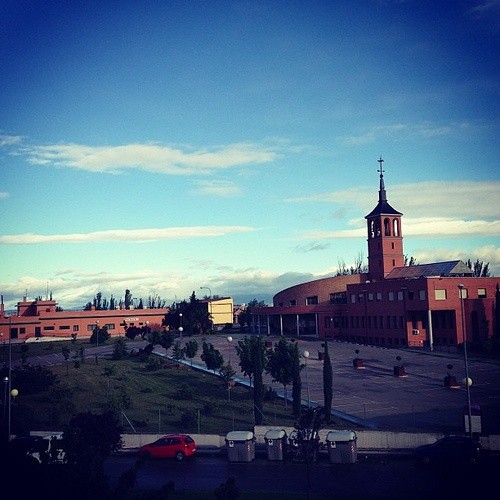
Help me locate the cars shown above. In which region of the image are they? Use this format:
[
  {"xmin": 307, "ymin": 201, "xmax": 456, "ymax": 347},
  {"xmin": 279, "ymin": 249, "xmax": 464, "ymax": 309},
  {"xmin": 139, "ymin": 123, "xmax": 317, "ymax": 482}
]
[{"xmin": 136, "ymin": 435, "xmax": 198, "ymax": 460}]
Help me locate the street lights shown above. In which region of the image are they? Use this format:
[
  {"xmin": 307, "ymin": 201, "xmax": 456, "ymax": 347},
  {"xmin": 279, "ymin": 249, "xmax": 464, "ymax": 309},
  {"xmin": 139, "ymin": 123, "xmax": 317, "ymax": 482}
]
[
  {"xmin": 145, "ymin": 320, "xmax": 149, "ymax": 347},
  {"xmin": 200, "ymin": 286, "xmax": 214, "ymax": 331},
  {"xmin": 227, "ymin": 337, "xmax": 232, "ymax": 365},
  {"xmin": 3, "ymin": 314, "xmax": 18, "ymax": 438},
  {"xmin": 458, "ymin": 283, "xmax": 473, "ymax": 438},
  {"xmin": 363, "ymin": 289, "xmax": 368, "ymax": 345},
  {"xmin": 96, "ymin": 320, "xmax": 99, "ymax": 347},
  {"xmin": 303, "ymin": 350, "xmax": 310, "ymax": 409},
  {"xmin": 401, "ymin": 286, "xmax": 408, "ymax": 348},
  {"xmin": 178, "ymin": 326, "xmax": 183, "ymax": 340}
]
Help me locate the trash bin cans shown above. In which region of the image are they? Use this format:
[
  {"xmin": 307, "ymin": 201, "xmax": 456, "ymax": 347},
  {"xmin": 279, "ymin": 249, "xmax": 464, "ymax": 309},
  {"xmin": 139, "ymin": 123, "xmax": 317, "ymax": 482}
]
[
  {"xmin": 264, "ymin": 428, "xmax": 286, "ymax": 461},
  {"xmin": 326, "ymin": 431, "xmax": 358, "ymax": 464},
  {"xmin": 288, "ymin": 430, "xmax": 317, "ymax": 461},
  {"xmin": 225, "ymin": 430, "xmax": 256, "ymax": 462}
]
